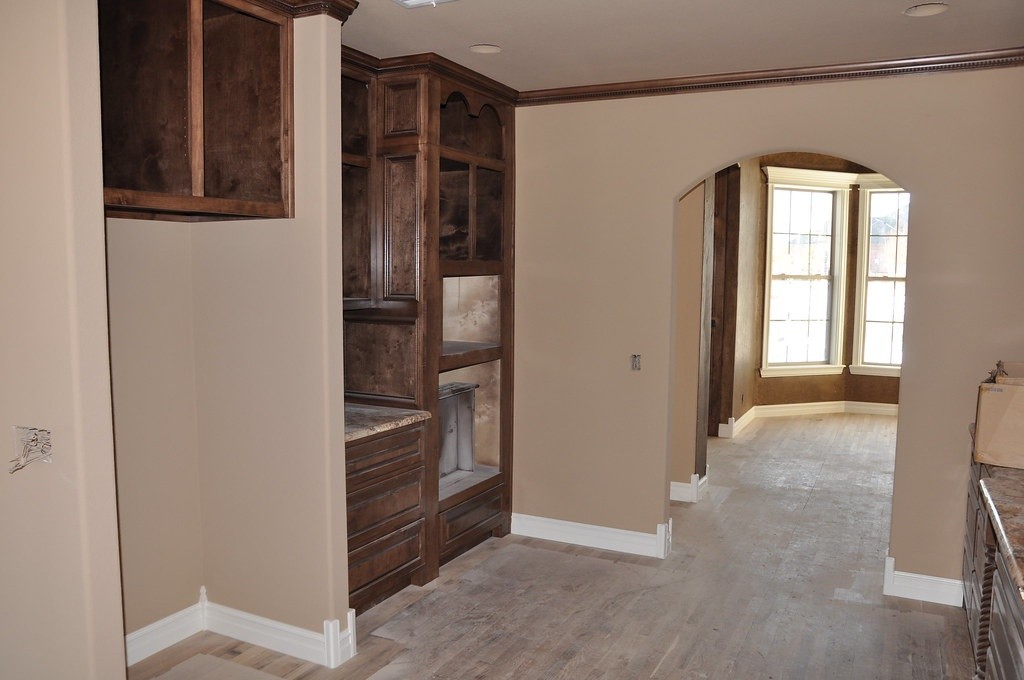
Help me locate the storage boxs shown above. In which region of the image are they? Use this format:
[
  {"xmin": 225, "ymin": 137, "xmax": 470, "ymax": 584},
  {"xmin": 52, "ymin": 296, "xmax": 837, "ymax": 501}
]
[{"xmin": 972, "ymin": 360, "xmax": 1024, "ymax": 470}]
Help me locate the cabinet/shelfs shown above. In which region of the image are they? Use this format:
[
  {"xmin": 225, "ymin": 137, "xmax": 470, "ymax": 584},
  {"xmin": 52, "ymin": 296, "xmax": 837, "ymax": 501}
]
[
  {"xmin": 961, "ymin": 424, "xmax": 1024, "ymax": 680},
  {"xmin": 341, "ymin": 44, "xmax": 519, "ymax": 617},
  {"xmin": 98, "ymin": 0, "xmax": 295, "ymax": 222}
]
